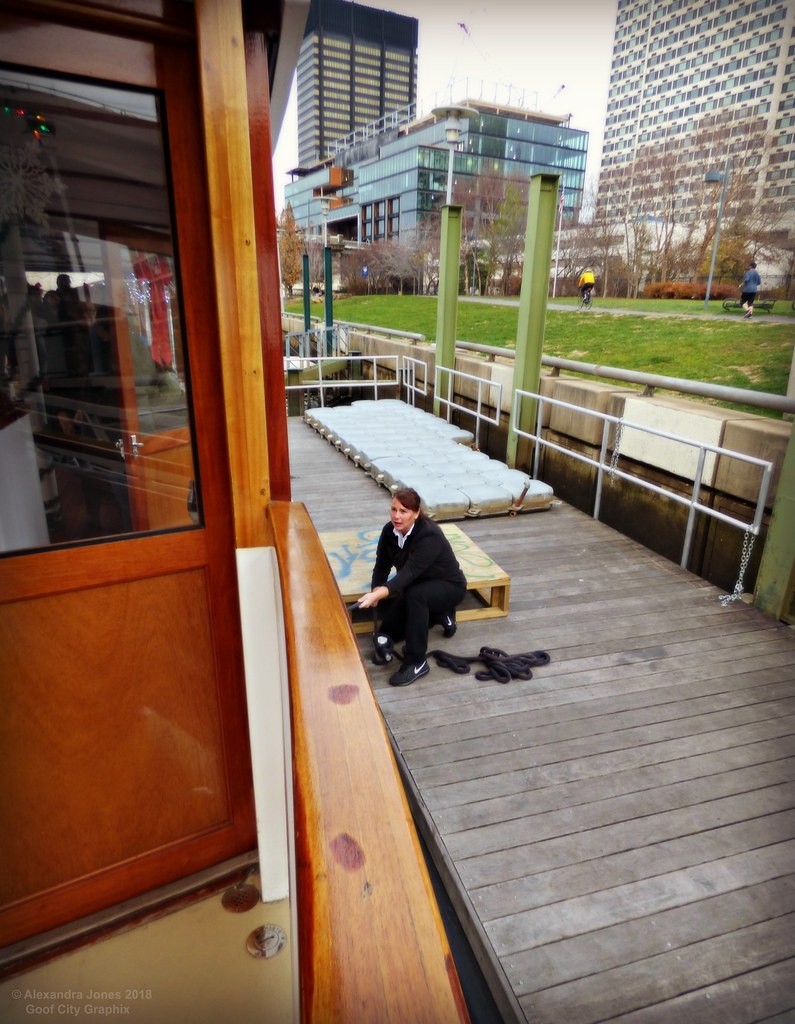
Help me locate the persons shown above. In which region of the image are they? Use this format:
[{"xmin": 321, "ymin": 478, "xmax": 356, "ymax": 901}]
[
  {"xmin": 577, "ymin": 268, "xmax": 595, "ymax": 305},
  {"xmin": 356, "ymin": 488, "xmax": 469, "ymax": 687},
  {"xmin": 738, "ymin": 262, "xmax": 762, "ymax": 318},
  {"xmin": 1, "ymin": 269, "xmax": 190, "ymax": 541},
  {"xmin": 313, "ymin": 285, "xmax": 323, "ymax": 298}
]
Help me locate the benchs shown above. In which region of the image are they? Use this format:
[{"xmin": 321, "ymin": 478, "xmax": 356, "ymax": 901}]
[{"xmin": 722, "ymin": 297, "xmax": 775, "ymax": 313}]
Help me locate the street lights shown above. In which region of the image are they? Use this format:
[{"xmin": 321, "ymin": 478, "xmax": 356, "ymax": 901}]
[
  {"xmin": 310, "ymin": 196, "xmax": 340, "ymax": 250},
  {"xmin": 430, "ymin": 106, "xmax": 481, "ymax": 206},
  {"xmin": 703, "ymin": 158, "xmax": 731, "ymax": 308}
]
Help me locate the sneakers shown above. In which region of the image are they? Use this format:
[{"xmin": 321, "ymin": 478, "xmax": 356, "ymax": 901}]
[
  {"xmin": 441, "ymin": 606, "xmax": 458, "ymax": 638},
  {"xmin": 389, "ymin": 657, "xmax": 430, "ymax": 686}
]
